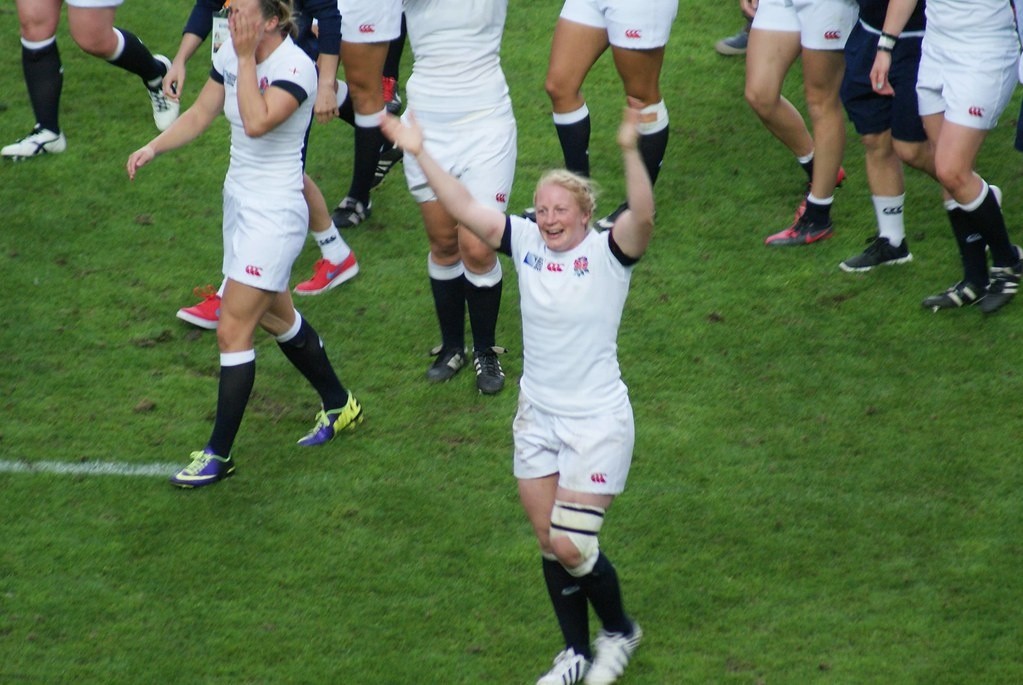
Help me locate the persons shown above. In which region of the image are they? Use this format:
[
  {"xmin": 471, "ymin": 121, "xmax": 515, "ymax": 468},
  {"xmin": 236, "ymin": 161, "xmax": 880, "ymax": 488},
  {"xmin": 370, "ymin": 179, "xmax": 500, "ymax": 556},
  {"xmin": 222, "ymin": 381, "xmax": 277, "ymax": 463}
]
[
  {"xmin": 126, "ymin": 1, "xmax": 364, "ymax": 488},
  {"xmin": 1, "ymin": 1, "xmax": 1023, "ymax": 393},
  {"xmin": 377, "ymin": 95, "xmax": 655, "ymax": 685}
]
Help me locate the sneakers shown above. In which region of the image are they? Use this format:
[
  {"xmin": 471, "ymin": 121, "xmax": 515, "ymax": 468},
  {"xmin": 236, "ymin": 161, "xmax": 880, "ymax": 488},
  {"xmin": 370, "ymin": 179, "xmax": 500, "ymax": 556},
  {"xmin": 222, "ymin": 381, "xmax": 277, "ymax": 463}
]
[
  {"xmin": 582, "ymin": 622, "xmax": 644, "ymax": 685},
  {"xmin": 922, "ymin": 281, "xmax": 992, "ymax": 310},
  {"xmin": 522, "ymin": 207, "xmax": 536, "ymax": 222},
  {"xmin": 144, "ymin": 54, "xmax": 180, "ymax": 131},
  {"xmin": 1, "ymin": 124, "xmax": 65, "ymax": 160},
  {"xmin": 382, "ymin": 76, "xmax": 402, "ymax": 114},
  {"xmin": 427, "ymin": 347, "xmax": 466, "ymax": 380},
  {"xmin": 714, "ymin": 31, "xmax": 749, "ymax": 55},
  {"xmin": 537, "ymin": 647, "xmax": 591, "ymax": 685},
  {"xmin": 839, "ymin": 237, "xmax": 913, "ymax": 272},
  {"xmin": 171, "ymin": 447, "xmax": 236, "ymax": 488},
  {"xmin": 370, "ymin": 142, "xmax": 405, "ymax": 189},
  {"xmin": 982, "ymin": 244, "xmax": 1023, "ymax": 312},
  {"xmin": 796, "ymin": 165, "xmax": 844, "ymax": 220},
  {"xmin": 297, "ymin": 389, "xmax": 363, "ymax": 446},
  {"xmin": 294, "ymin": 250, "xmax": 361, "ymax": 295},
  {"xmin": 598, "ymin": 204, "xmax": 659, "ymax": 228},
  {"xmin": 331, "ymin": 197, "xmax": 371, "ymax": 227},
  {"xmin": 764, "ymin": 217, "xmax": 833, "ymax": 246},
  {"xmin": 473, "ymin": 349, "xmax": 504, "ymax": 394},
  {"xmin": 176, "ymin": 285, "xmax": 222, "ymax": 329}
]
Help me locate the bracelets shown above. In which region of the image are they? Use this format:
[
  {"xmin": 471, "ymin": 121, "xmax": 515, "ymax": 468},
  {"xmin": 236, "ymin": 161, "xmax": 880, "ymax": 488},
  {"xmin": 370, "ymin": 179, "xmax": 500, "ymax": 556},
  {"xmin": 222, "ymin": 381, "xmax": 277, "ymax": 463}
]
[{"xmin": 877, "ymin": 33, "xmax": 898, "ymax": 54}]
